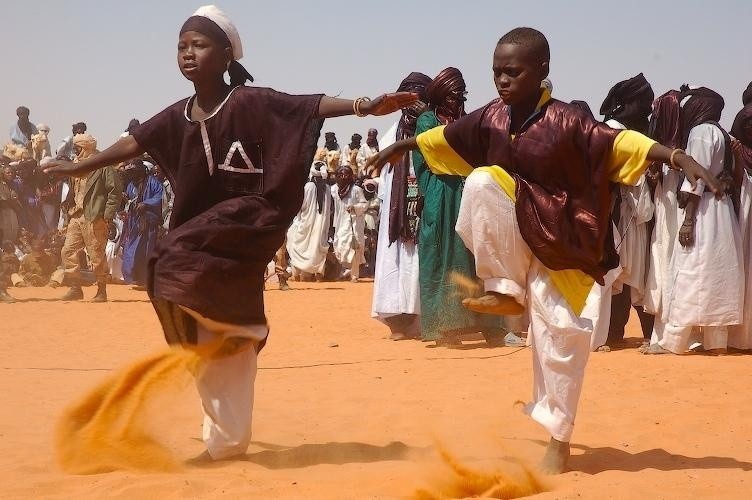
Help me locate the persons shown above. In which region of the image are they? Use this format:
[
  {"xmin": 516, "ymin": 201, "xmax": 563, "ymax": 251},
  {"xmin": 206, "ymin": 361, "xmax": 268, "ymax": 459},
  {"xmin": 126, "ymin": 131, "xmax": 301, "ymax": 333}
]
[
  {"xmin": 362, "ymin": 26, "xmax": 727, "ymax": 476},
  {"xmin": 263, "ymin": 127, "xmax": 380, "ymax": 290},
  {"xmin": 0, "ymin": 106, "xmax": 175, "ymax": 303},
  {"xmin": 570, "ymin": 72, "xmax": 752, "ymax": 354},
  {"xmin": 379, "ymin": 66, "xmax": 529, "ymax": 347},
  {"xmin": 40, "ymin": 5, "xmax": 420, "ymax": 471}
]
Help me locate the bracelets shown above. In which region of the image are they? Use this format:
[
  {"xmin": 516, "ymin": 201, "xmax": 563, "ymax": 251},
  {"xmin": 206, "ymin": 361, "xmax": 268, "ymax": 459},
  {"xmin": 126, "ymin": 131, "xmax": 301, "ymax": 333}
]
[
  {"xmin": 670, "ymin": 144, "xmax": 686, "ymax": 170},
  {"xmin": 354, "ymin": 97, "xmax": 372, "ymax": 119}
]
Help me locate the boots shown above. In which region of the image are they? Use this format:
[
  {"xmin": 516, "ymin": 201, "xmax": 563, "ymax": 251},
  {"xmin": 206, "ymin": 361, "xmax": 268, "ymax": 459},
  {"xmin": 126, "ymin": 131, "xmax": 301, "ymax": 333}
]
[
  {"xmin": 279, "ymin": 275, "xmax": 289, "ymax": 290},
  {"xmin": 60, "ymin": 281, "xmax": 83, "ymax": 301},
  {"xmin": 91, "ymin": 282, "xmax": 107, "ymax": 301}
]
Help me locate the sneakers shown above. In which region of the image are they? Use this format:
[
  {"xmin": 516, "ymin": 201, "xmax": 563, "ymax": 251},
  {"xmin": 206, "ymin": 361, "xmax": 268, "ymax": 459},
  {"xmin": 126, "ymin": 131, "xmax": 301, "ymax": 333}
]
[{"xmin": 0, "ymin": 287, "xmax": 17, "ymax": 303}]
